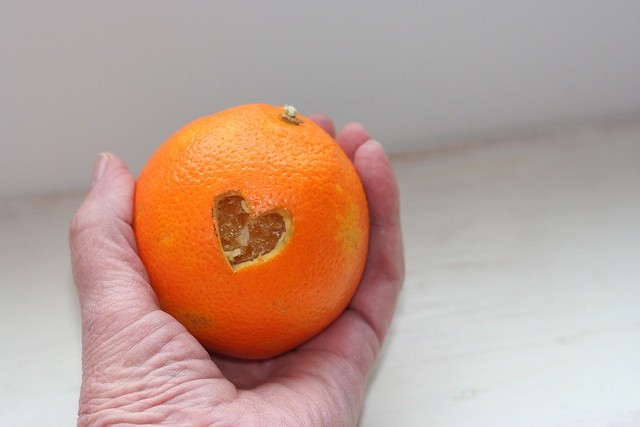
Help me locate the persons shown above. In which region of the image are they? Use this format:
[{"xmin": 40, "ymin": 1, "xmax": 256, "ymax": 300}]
[{"xmin": 68, "ymin": 113, "xmax": 407, "ymax": 427}]
